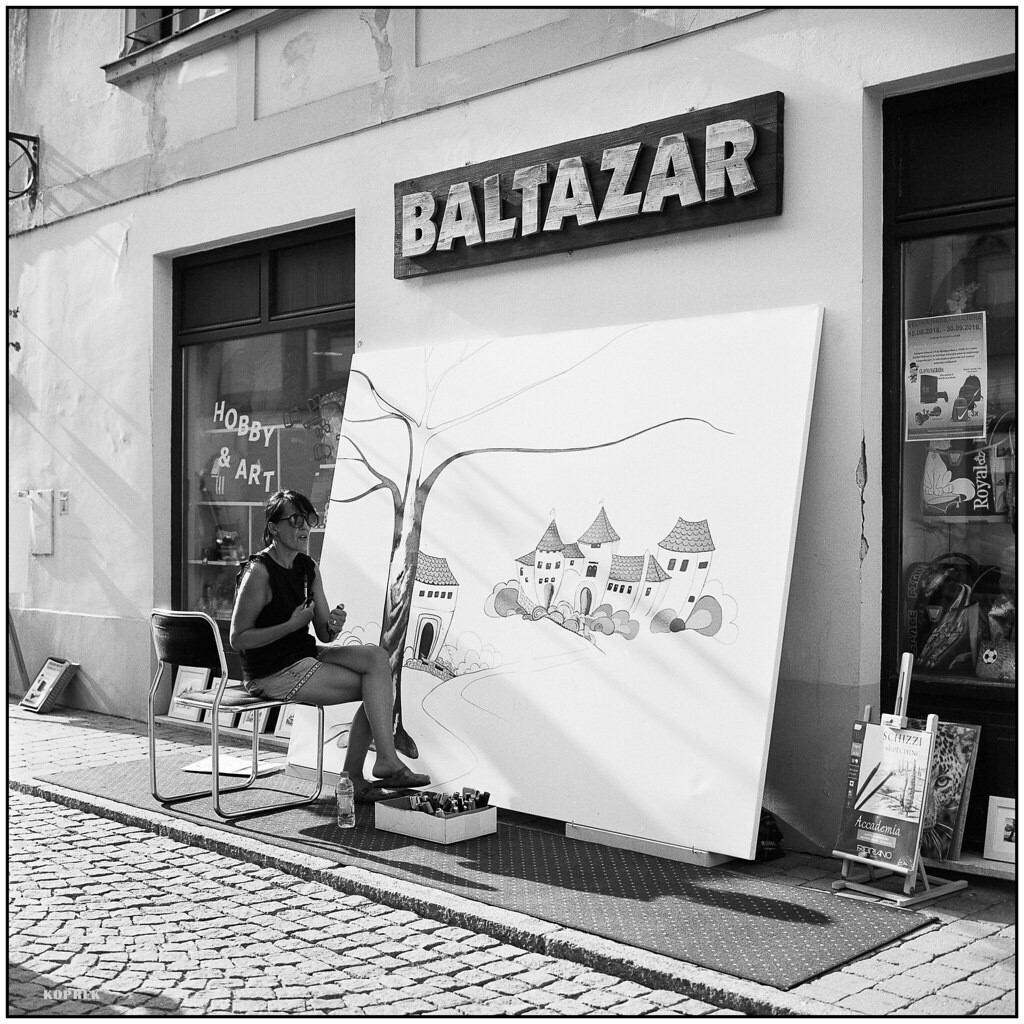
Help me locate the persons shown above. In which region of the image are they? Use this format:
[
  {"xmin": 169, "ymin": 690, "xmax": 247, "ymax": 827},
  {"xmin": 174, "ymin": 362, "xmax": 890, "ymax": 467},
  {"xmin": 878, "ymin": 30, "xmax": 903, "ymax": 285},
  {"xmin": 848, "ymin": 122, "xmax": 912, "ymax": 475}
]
[{"xmin": 229, "ymin": 490, "xmax": 431, "ymax": 806}]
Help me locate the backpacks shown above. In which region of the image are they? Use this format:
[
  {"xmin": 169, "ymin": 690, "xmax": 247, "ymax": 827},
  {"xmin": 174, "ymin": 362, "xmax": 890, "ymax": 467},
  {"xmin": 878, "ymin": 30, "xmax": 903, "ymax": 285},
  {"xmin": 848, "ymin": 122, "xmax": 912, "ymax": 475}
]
[
  {"xmin": 952, "ymin": 398, "xmax": 968, "ymax": 422},
  {"xmin": 965, "ymin": 375, "xmax": 981, "ymax": 401},
  {"xmin": 959, "ymin": 385, "xmax": 974, "ymax": 410}
]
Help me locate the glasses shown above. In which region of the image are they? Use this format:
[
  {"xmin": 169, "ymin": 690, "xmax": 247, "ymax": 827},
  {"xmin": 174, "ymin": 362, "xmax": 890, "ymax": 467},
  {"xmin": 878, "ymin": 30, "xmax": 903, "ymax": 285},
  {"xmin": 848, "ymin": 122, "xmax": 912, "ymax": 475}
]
[{"xmin": 276, "ymin": 514, "xmax": 319, "ymax": 529}]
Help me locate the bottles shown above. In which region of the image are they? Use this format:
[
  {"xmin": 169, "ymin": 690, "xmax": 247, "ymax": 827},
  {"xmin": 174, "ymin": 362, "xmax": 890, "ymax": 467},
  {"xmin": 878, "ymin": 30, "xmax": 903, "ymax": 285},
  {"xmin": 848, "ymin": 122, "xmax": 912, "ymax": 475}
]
[{"xmin": 336, "ymin": 771, "xmax": 355, "ymax": 828}]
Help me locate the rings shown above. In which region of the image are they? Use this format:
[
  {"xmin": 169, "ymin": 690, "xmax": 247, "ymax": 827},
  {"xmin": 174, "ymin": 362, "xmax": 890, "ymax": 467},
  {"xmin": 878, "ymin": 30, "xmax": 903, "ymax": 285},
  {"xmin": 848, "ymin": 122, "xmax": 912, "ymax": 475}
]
[{"xmin": 334, "ymin": 621, "xmax": 336, "ymax": 623}]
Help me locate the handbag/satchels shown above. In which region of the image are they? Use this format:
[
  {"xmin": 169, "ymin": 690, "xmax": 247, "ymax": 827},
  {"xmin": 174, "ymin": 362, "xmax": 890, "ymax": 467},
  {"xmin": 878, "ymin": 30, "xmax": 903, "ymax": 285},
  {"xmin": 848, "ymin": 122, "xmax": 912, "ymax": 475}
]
[
  {"xmin": 905, "ymin": 549, "xmax": 1017, "ymax": 683},
  {"xmin": 918, "ymin": 410, "xmax": 1015, "ymax": 523},
  {"xmin": 928, "ymin": 259, "xmax": 997, "ymax": 324}
]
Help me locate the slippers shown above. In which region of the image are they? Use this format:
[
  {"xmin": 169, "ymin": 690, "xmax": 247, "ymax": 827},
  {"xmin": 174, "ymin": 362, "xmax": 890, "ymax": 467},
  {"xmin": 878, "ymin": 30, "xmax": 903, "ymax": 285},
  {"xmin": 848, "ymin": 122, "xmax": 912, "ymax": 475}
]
[
  {"xmin": 372, "ymin": 766, "xmax": 431, "ymax": 787},
  {"xmin": 335, "ymin": 781, "xmax": 400, "ymax": 804}
]
